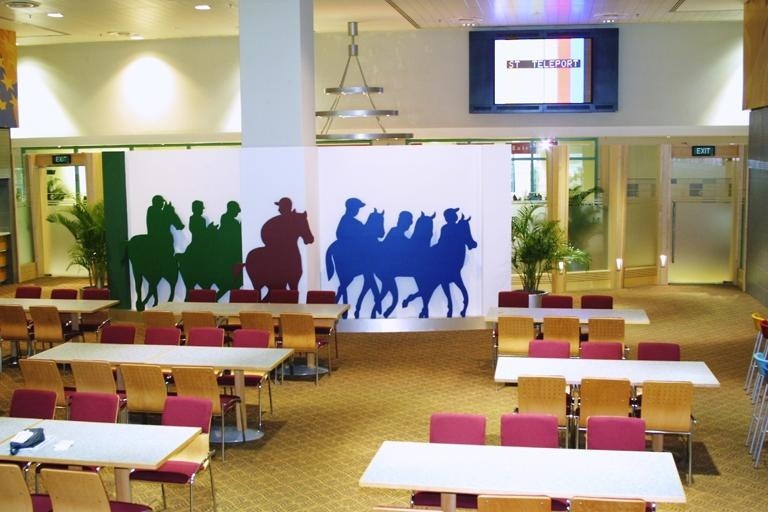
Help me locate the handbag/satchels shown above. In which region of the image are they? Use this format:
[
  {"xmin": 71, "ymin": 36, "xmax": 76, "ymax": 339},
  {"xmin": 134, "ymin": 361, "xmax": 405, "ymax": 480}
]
[{"xmin": 9, "ymin": 428, "xmax": 44, "ymax": 455}]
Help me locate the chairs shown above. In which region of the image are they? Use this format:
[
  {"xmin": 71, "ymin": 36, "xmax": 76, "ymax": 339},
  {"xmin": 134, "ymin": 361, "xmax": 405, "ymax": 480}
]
[
  {"xmin": 476, "ymin": 494, "xmax": 552, "ymax": 510},
  {"xmin": 741, "ymin": 308, "xmax": 767, "ymax": 467},
  {"xmin": 570, "ymin": 497, "xmax": 645, "ymax": 511},
  {"xmin": 635, "ymin": 380, "xmax": 697, "ymax": 487},
  {"xmin": 574, "ymin": 379, "xmax": 630, "ymax": 450},
  {"xmin": 410, "ymin": 414, "xmax": 487, "ymax": 509},
  {"xmin": 119, "ymin": 361, "xmax": 168, "ymax": 423},
  {"xmin": 587, "ymin": 318, "xmax": 626, "ymax": 359},
  {"xmin": 128, "ymin": 396, "xmax": 216, "ymax": 511},
  {"xmin": 31, "ymin": 391, "xmax": 120, "ymax": 495},
  {"xmin": 278, "ymin": 313, "xmax": 331, "ymax": 388},
  {"xmin": 490, "ymin": 289, "xmax": 531, "ymax": 370},
  {"xmin": 528, "ymin": 340, "xmax": 574, "ymax": 415},
  {"xmin": 41, "ymin": 468, "xmax": 152, "ymax": 511},
  {"xmin": 71, "ymin": 359, "xmax": 126, "ymax": 423},
  {"xmin": 0, "ymin": 389, "xmax": 58, "ymax": 484},
  {"xmin": 145, "ymin": 326, "xmax": 181, "ymax": 384},
  {"xmin": 633, "ymin": 341, "xmax": 682, "ymax": 418},
  {"xmin": 1, "ymin": 464, "xmax": 52, "ymax": 511},
  {"xmin": 534, "ymin": 295, "xmax": 574, "ymax": 338},
  {"xmin": 585, "ymin": 415, "xmax": 655, "ymax": 512},
  {"xmin": 501, "ymin": 415, "xmax": 569, "ymax": 509},
  {"xmin": 238, "ymin": 312, "xmax": 280, "ymax": 384},
  {"xmin": 496, "ymin": 316, "xmax": 536, "ymax": 357},
  {"xmin": 518, "ymin": 376, "xmax": 572, "ymax": 448},
  {"xmin": 18, "ymin": 358, "xmax": 76, "ymax": 421},
  {"xmin": 578, "ymin": 295, "xmax": 613, "ymax": 344},
  {"xmin": 186, "ymin": 328, "xmax": 229, "ymax": 395},
  {"xmin": 1, "ymin": 283, "xmax": 349, "ymax": 366},
  {"xmin": 170, "ymin": 366, "xmax": 247, "ymax": 463},
  {"xmin": 215, "ymin": 330, "xmax": 274, "ymax": 430},
  {"xmin": 576, "ymin": 343, "xmax": 631, "ymax": 405},
  {"xmin": 542, "ymin": 318, "xmax": 580, "ymax": 359}
]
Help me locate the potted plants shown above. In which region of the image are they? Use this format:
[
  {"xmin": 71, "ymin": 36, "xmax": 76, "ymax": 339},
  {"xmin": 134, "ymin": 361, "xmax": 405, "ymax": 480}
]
[
  {"xmin": 510, "ymin": 201, "xmax": 591, "ymax": 309},
  {"xmin": 45, "ymin": 192, "xmax": 113, "ymax": 299}
]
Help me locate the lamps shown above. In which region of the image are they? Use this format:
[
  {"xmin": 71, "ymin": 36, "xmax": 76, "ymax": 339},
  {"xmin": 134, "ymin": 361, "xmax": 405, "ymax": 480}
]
[{"xmin": 314, "ymin": 21, "xmax": 412, "ymax": 141}]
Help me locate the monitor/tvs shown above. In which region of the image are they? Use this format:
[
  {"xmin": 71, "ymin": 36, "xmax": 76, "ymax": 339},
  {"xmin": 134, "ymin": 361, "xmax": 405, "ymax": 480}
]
[{"xmin": 492, "ymin": 35, "xmax": 592, "ymax": 104}]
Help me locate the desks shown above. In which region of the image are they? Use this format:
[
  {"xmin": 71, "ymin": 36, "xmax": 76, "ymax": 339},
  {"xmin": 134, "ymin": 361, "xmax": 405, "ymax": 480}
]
[
  {"xmin": 27, "ymin": 342, "xmax": 296, "ymax": 446},
  {"xmin": 2, "ymin": 418, "xmax": 203, "ymax": 504},
  {"xmin": 492, "ymin": 356, "xmax": 721, "ymax": 452},
  {"xmin": 359, "ymin": 440, "xmax": 689, "ymax": 510},
  {"xmin": 494, "ymin": 304, "xmax": 651, "ymax": 342}
]
